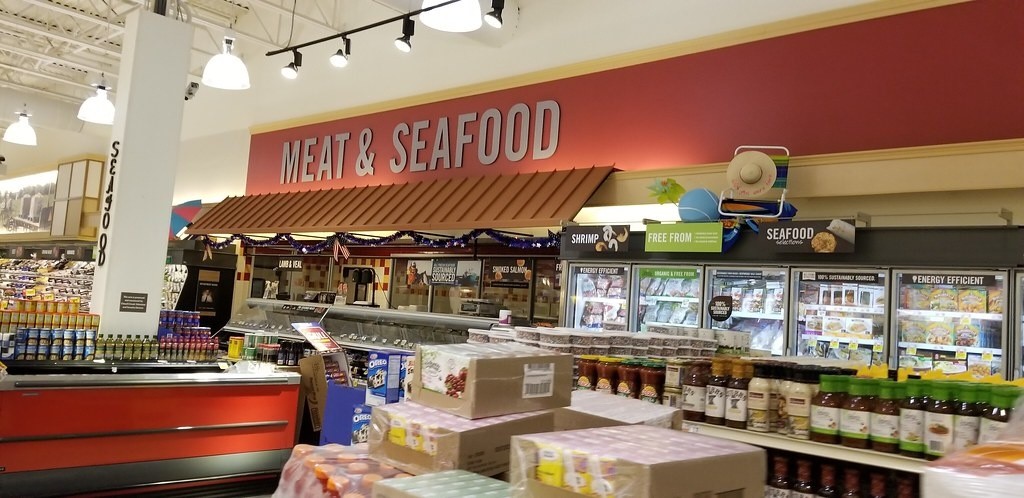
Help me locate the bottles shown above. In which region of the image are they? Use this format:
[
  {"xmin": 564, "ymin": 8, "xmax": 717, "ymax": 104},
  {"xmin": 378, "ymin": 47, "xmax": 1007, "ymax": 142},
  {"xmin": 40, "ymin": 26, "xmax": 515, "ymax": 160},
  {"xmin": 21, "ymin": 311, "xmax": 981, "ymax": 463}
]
[
  {"xmin": 682, "ymin": 355, "xmax": 1024, "ymax": 468},
  {"xmin": 765, "ymin": 457, "xmax": 917, "ymax": 498},
  {"xmin": 95, "ymin": 334, "xmax": 219, "ymax": 362}
]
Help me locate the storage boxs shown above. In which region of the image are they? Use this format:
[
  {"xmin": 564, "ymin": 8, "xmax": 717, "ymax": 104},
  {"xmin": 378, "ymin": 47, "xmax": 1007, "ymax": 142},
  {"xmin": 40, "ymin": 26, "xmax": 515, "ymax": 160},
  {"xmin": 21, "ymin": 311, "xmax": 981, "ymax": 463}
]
[
  {"xmin": 320, "ymin": 349, "xmax": 416, "ymax": 446},
  {"xmin": 300, "ymin": 352, "xmax": 354, "ymax": 432},
  {"xmin": 367, "ymin": 341, "xmax": 767, "ymax": 497}
]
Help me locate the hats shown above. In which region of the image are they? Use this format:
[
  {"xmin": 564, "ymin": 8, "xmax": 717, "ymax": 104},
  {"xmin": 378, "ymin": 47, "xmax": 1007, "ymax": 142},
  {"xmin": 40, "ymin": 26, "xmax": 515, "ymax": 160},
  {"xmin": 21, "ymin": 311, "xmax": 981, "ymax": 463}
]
[{"xmin": 727, "ymin": 151, "xmax": 776, "ymax": 198}]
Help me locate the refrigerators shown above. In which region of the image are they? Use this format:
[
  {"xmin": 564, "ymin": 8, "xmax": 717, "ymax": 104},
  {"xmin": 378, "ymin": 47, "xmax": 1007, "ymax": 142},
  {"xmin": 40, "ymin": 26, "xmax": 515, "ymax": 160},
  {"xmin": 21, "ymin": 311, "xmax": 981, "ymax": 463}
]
[
  {"xmin": 788, "ymin": 264, "xmax": 890, "ymax": 366},
  {"xmin": 704, "ymin": 265, "xmax": 788, "ymax": 354},
  {"xmin": 890, "ymin": 266, "xmax": 1008, "ymax": 382},
  {"xmin": 629, "ymin": 263, "xmax": 703, "ymax": 333},
  {"xmin": 558, "ymin": 260, "xmax": 627, "ymax": 332},
  {"xmin": 1012, "ymin": 269, "xmax": 1024, "ymax": 385}
]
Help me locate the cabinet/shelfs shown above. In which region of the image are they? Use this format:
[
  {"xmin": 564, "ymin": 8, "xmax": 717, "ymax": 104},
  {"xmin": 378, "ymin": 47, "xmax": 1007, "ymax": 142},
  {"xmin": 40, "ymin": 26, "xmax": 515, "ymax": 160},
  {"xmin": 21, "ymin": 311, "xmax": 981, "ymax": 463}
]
[{"xmin": 0, "ymin": 258, "xmax": 188, "ymax": 314}]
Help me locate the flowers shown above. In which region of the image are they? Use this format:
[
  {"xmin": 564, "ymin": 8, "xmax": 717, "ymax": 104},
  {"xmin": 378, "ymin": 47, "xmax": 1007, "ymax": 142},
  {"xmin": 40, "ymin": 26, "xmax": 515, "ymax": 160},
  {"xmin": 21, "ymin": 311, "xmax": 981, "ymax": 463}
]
[{"xmin": 201, "ymin": 227, "xmax": 562, "ymax": 255}]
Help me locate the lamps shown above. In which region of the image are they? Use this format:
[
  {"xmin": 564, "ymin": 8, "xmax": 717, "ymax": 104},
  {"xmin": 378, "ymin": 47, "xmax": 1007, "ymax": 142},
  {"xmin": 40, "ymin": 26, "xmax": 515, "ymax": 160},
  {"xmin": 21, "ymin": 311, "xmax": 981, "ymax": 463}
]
[
  {"xmin": 76, "ymin": 77, "xmax": 115, "ymax": 125},
  {"xmin": 484, "ymin": 0, "xmax": 505, "ymax": 28},
  {"xmin": 3, "ymin": 107, "xmax": 37, "ymax": 146},
  {"xmin": 200, "ymin": 28, "xmax": 250, "ymax": 91},
  {"xmin": 282, "ymin": 48, "xmax": 302, "ymax": 80},
  {"xmin": 330, "ymin": 34, "xmax": 350, "ymax": 68},
  {"xmin": 395, "ymin": 14, "xmax": 415, "ymax": 52}
]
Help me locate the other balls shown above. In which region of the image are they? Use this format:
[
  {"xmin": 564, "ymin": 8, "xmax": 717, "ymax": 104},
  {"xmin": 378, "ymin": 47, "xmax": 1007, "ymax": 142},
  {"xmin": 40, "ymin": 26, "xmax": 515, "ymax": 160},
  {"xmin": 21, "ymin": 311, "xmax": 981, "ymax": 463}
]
[{"xmin": 678, "ymin": 187, "xmax": 721, "ymax": 223}]
